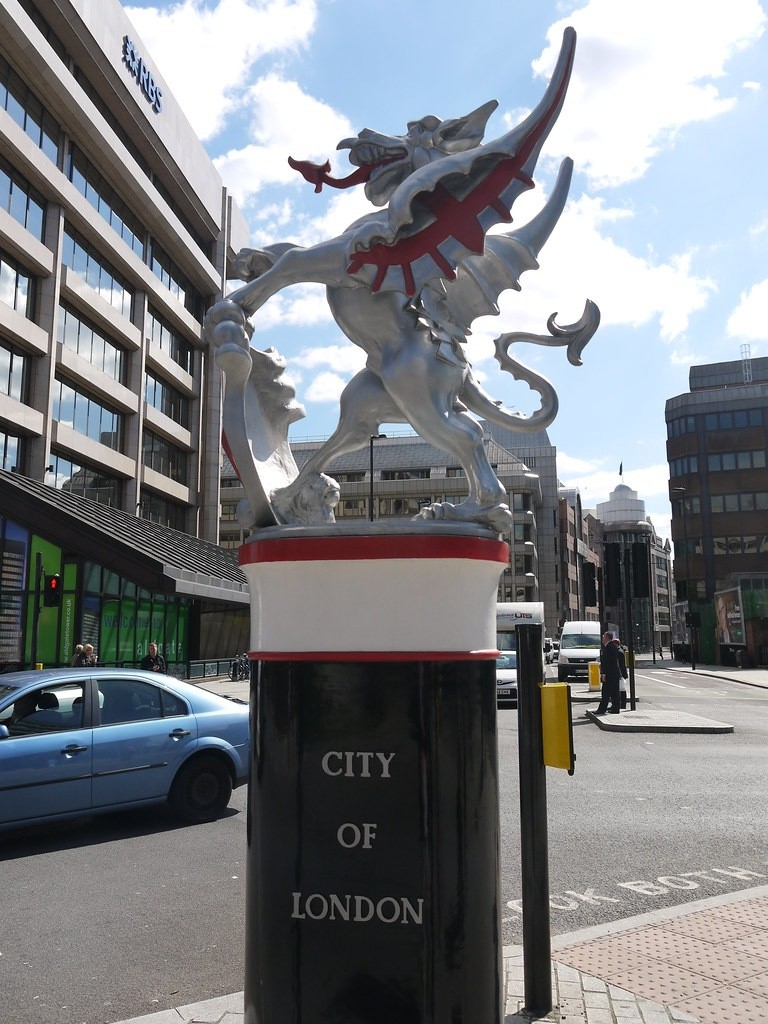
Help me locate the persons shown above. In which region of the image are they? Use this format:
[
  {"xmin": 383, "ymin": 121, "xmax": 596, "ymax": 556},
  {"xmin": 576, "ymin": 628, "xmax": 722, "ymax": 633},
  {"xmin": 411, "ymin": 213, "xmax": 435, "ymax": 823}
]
[
  {"xmin": 658, "ymin": 645, "xmax": 663, "ymax": 660},
  {"xmin": 71, "ymin": 644, "xmax": 96, "ymax": 668},
  {"xmin": 717, "ymin": 597, "xmax": 734, "ymax": 643},
  {"xmin": 592, "ymin": 632, "xmax": 628, "ymax": 715},
  {"xmin": 140, "ymin": 643, "xmax": 165, "ymax": 674}
]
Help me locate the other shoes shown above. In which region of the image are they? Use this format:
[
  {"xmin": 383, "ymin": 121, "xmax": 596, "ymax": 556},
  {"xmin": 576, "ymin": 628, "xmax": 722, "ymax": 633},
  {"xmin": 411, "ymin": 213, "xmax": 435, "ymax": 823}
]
[
  {"xmin": 606, "ymin": 707, "xmax": 619, "ymax": 714},
  {"xmin": 592, "ymin": 711, "xmax": 606, "ymax": 715},
  {"xmin": 620, "ymin": 705, "xmax": 627, "ymax": 709}
]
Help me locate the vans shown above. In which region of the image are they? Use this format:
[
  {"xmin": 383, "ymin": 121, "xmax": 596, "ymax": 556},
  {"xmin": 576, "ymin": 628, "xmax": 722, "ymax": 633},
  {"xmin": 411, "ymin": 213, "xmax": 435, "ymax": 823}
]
[{"xmin": 543, "ymin": 638, "xmax": 554, "ymax": 664}]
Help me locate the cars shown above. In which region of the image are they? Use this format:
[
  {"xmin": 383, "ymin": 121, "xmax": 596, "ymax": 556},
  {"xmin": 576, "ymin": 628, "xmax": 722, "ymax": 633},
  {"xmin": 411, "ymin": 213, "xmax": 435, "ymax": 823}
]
[
  {"xmin": 495, "ymin": 651, "xmax": 517, "ymax": 708},
  {"xmin": 0, "ymin": 667, "xmax": 250, "ymax": 830},
  {"xmin": 552, "ymin": 642, "xmax": 560, "ymax": 660}
]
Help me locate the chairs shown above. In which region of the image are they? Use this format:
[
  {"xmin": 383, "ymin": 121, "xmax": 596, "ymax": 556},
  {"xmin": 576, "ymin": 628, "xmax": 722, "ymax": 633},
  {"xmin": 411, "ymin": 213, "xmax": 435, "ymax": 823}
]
[
  {"xmin": 64, "ymin": 697, "xmax": 83, "ymax": 728},
  {"xmin": 36, "ymin": 692, "xmax": 64, "ymax": 724}
]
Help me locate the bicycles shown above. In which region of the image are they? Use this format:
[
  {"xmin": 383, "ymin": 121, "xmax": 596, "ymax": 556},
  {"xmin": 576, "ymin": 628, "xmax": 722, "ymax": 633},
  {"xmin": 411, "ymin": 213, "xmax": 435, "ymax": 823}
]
[{"xmin": 228, "ymin": 654, "xmax": 250, "ymax": 681}]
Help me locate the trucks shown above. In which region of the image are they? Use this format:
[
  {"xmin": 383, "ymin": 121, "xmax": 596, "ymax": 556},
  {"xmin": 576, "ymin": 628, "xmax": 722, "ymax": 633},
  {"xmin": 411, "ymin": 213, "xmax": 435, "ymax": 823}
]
[
  {"xmin": 558, "ymin": 621, "xmax": 619, "ymax": 681},
  {"xmin": 496, "ymin": 601, "xmax": 546, "ymax": 685}
]
